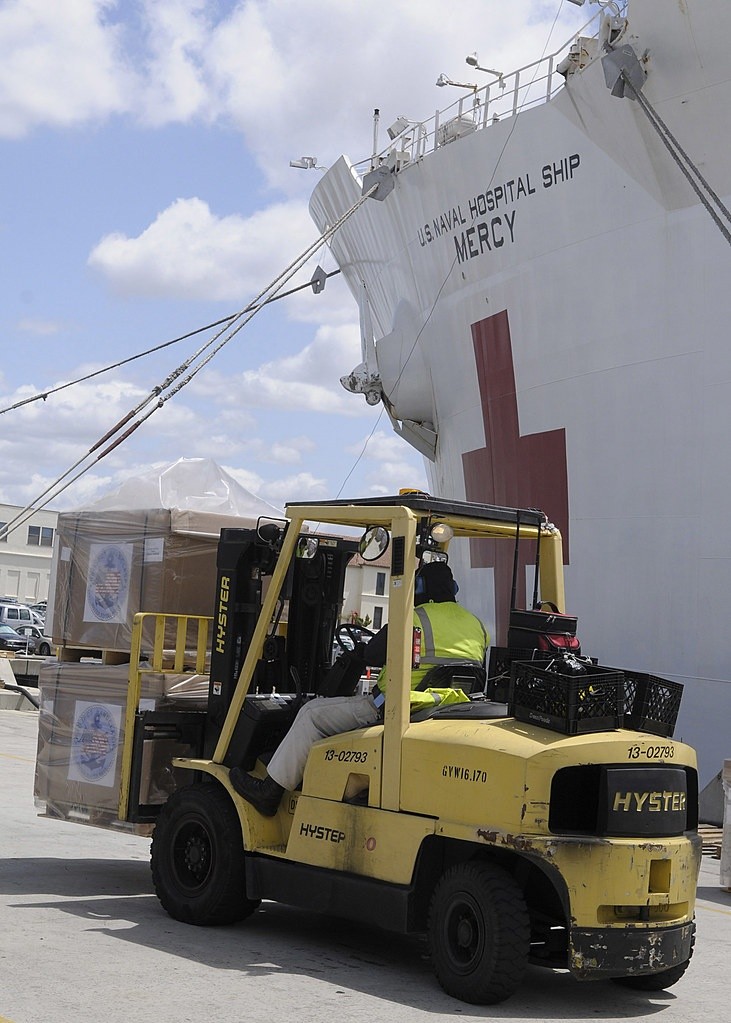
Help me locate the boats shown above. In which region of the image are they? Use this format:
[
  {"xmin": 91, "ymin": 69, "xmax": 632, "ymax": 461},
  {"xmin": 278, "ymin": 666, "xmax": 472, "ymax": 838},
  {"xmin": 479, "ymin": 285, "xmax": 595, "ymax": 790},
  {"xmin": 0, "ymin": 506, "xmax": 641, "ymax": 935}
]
[{"xmin": 287, "ymin": 0, "xmax": 731, "ymax": 835}]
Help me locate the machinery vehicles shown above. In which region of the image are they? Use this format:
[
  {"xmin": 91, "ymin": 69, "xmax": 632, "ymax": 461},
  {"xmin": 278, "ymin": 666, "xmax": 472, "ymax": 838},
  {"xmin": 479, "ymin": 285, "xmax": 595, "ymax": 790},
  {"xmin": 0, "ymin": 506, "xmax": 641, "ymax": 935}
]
[{"xmin": 118, "ymin": 486, "xmax": 704, "ymax": 1008}]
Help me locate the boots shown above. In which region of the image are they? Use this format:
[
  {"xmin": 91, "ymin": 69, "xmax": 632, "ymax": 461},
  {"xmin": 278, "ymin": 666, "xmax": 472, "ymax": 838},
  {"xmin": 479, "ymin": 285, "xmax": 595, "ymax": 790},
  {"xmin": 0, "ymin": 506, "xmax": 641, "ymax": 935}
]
[{"xmin": 229, "ymin": 766, "xmax": 286, "ymax": 817}]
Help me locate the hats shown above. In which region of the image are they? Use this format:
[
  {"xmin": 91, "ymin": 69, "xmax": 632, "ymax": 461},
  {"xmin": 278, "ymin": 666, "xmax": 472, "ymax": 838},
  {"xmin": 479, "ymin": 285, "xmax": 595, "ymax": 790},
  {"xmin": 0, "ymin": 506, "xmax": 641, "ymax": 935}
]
[{"xmin": 413, "ymin": 561, "xmax": 459, "ymax": 607}]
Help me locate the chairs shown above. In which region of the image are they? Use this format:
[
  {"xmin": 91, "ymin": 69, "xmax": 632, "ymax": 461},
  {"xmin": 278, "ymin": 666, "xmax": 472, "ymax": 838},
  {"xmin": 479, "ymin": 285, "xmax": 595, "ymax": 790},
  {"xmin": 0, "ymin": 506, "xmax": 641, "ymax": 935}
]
[{"xmin": 417, "ymin": 658, "xmax": 487, "ymax": 702}]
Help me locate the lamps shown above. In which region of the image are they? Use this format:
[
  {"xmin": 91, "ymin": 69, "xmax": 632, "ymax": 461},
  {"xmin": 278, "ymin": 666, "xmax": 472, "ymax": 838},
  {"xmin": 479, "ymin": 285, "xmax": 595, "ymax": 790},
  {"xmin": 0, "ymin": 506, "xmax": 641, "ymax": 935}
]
[
  {"xmin": 289, "ymin": 156, "xmax": 329, "ymax": 172},
  {"xmin": 466, "ymin": 52, "xmax": 506, "ymax": 87},
  {"xmin": 568, "ymin": 0, "xmax": 610, "ymax": 8},
  {"xmin": 435, "ymin": 73, "xmax": 478, "ymax": 90},
  {"xmin": 386, "ymin": 114, "xmax": 429, "ymax": 138}
]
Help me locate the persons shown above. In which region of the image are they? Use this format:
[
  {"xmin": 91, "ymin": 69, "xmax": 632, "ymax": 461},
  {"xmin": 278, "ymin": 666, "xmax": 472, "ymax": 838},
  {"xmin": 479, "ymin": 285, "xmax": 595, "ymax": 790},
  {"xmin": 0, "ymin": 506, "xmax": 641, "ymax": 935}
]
[
  {"xmin": 362, "ymin": 535, "xmax": 366, "ymax": 552},
  {"xmin": 230, "ymin": 562, "xmax": 489, "ymax": 816},
  {"xmin": 297, "ymin": 538, "xmax": 307, "ymax": 557}
]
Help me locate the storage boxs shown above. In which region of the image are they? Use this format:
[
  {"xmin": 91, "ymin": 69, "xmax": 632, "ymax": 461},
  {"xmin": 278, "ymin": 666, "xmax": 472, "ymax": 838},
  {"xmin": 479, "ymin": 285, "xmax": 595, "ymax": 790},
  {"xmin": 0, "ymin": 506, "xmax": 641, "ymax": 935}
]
[
  {"xmin": 486, "ymin": 646, "xmax": 685, "ymax": 742},
  {"xmin": 30, "ymin": 507, "xmax": 308, "ymax": 810}
]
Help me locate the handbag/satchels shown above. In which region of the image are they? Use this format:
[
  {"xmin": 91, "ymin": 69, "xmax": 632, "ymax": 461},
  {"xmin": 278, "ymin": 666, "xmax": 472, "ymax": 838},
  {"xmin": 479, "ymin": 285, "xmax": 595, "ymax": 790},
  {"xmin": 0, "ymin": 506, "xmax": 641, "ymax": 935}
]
[{"xmin": 508, "ymin": 601, "xmax": 581, "ymax": 656}]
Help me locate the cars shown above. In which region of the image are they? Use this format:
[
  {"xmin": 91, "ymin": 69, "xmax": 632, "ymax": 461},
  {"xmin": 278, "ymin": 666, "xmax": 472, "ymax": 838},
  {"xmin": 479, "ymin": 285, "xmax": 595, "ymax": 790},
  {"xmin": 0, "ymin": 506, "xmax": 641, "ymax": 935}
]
[
  {"xmin": 330, "ymin": 627, "xmax": 383, "ymax": 680},
  {"xmin": 0, "ymin": 595, "xmax": 59, "ymax": 659}
]
